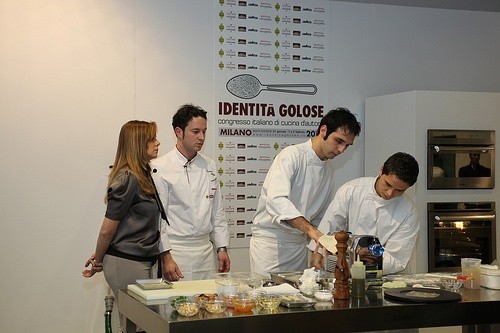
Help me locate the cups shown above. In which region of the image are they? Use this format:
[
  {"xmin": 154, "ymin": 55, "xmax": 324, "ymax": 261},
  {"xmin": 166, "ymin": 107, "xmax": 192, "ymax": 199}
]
[{"xmin": 460, "ymin": 258, "xmax": 482, "ymax": 290}]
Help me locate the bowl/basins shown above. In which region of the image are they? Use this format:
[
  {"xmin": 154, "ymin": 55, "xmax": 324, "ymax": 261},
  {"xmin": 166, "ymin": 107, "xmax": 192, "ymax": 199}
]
[
  {"xmin": 314, "ymin": 289, "xmax": 333, "ymax": 301},
  {"xmin": 169, "ymin": 290, "xmax": 283, "ymax": 317}
]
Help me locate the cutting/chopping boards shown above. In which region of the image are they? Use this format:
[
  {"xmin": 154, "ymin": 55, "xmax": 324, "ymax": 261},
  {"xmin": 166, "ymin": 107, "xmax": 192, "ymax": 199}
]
[{"xmin": 126, "ymin": 278, "xmax": 253, "ymax": 301}]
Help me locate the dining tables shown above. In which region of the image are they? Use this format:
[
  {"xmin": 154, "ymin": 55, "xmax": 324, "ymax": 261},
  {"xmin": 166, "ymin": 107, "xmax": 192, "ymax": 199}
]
[{"xmin": 119, "ymin": 273, "xmax": 500, "ymax": 333}]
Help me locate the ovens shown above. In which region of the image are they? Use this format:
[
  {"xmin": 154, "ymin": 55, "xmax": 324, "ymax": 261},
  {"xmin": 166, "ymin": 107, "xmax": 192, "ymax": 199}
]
[
  {"xmin": 426, "ymin": 129, "xmax": 496, "ymax": 190},
  {"xmin": 427, "ymin": 201, "xmax": 497, "ymax": 273}
]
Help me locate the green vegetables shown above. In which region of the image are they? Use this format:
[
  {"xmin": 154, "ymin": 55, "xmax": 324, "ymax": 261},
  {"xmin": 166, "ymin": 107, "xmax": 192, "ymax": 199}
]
[{"xmin": 171, "ymin": 295, "xmax": 188, "ymax": 309}]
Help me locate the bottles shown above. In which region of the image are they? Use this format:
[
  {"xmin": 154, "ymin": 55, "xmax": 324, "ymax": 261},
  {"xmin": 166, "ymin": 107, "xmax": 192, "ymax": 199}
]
[{"xmin": 351, "ymin": 255, "xmax": 365, "ymax": 298}]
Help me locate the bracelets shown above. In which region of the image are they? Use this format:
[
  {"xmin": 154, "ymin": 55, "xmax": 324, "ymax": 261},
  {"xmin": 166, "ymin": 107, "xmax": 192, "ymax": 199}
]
[
  {"xmin": 217, "ymin": 248, "xmax": 227, "ymax": 253},
  {"xmin": 310, "ymin": 259, "xmax": 321, "ymax": 262}
]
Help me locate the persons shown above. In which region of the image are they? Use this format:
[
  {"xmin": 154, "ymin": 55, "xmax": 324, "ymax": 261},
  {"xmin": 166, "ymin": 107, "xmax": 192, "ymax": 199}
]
[
  {"xmin": 307, "ymin": 152, "xmax": 420, "ymax": 275},
  {"xmin": 458, "ymin": 152, "xmax": 490, "ymax": 226},
  {"xmin": 250, "ymin": 108, "xmax": 361, "ymax": 271},
  {"xmin": 147, "ymin": 103, "xmax": 231, "ymax": 281},
  {"xmin": 80, "ymin": 120, "xmax": 171, "ymax": 333}
]
